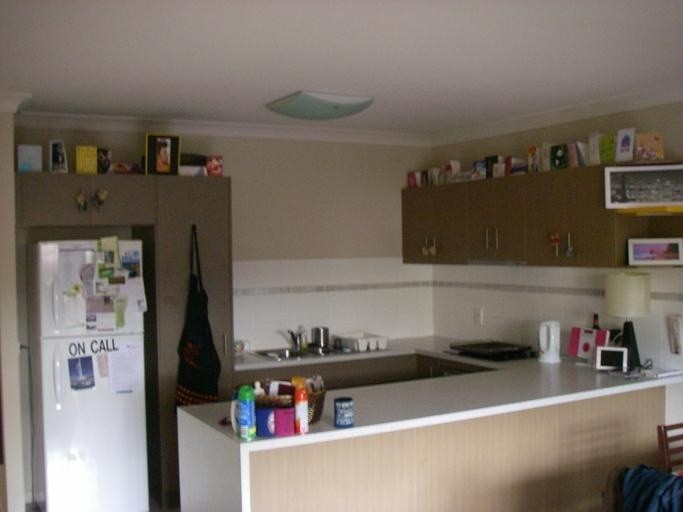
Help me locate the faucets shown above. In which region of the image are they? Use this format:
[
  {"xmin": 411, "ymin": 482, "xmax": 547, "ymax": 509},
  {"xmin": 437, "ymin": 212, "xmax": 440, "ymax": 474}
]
[{"xmin": 286, "ymin": 328, "xmax": 301, "ymax": 352}]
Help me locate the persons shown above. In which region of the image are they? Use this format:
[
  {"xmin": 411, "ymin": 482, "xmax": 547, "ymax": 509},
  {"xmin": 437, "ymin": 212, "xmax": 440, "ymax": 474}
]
[{"xmin": 156, "ymin": 140, "xmax": 170, "ymax": 172}]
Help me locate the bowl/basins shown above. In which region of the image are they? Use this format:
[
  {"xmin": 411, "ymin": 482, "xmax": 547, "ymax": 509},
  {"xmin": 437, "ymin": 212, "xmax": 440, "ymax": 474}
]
[{"xmin": 308, "ymin": 388, "xmax": 326, "ymax": 423}]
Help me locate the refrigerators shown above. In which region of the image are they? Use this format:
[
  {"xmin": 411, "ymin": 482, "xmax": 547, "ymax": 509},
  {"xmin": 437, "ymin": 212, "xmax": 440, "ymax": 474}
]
[{"xmin": 25, "ymin": 237, "xmax": 152, "ymax": 512}]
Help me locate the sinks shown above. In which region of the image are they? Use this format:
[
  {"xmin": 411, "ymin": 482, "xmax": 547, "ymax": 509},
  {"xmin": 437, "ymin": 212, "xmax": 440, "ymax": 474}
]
[{"xmin": 249, "ymin": 345, "xmax": 324, "ymax": 361}]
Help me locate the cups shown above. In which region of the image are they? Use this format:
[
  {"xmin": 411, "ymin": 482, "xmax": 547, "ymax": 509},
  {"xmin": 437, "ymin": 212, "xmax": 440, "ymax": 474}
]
[
  {"xmin": 312, "ymin": 325, "xmax": 331, "ymax": 354},
  {"xmin": 332, "ymin": 396, "xmax": 354, "ymax": 429}
]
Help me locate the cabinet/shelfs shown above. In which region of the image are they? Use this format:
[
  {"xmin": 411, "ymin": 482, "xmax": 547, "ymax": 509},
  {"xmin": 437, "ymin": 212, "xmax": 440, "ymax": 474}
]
[
  {"xmin": 156, "ymin": 176, "xmax": 234, "ymax": 512},
  {"xmin": 401, "ymin": 168, "xmax": 614, "ymax": 267},
  {"xmin": 22, "ymin": 173, "xmax": 157, "ymax": 227},
  {"xmin": 614, "ymin": 211, "xmax": 683, "ymax": 268}
]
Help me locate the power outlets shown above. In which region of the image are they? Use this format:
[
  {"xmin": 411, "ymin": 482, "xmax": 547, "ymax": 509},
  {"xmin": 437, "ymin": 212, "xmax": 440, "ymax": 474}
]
[{"xmin": 17, "ymin": 340, "xmax": 24, "ymax": 355}]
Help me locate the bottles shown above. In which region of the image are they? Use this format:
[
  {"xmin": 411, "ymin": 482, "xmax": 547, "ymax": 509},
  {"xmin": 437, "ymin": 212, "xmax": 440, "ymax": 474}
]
[
  {"xmin": 252, "ymin": 394, "xmax": 296, "ymax": 438},
  {"xmin": 298, "ymin": 325, "xmax": 309, "ymax": 355},
  {"xmin": 236, "ymin": 384, "xmax": 257, "ymax": 442},
  {"xmin": 294, "ymin": 384, "xmax": 310, "ymax": 436}
]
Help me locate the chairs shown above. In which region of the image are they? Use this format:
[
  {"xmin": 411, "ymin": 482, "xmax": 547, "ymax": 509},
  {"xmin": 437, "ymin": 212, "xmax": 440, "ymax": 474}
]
[{"xmin": 655, "ymin": 421, "xmax": 683, "ymax": 473}]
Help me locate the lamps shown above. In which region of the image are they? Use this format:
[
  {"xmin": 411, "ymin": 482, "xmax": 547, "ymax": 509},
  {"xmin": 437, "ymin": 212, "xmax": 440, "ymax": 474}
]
[
  {"xmin": 602, "ymin": 272, "xmax": 653, "ymax": 371},
  {"xmin": 264, "ymin": 89, "xmax": 374, "ymax": 121}
]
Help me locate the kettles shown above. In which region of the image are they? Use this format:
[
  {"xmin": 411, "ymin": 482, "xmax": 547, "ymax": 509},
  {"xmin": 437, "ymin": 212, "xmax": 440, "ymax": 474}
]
[{"xmin": 537, "ymin": 317, "xmax": 564, "ymax": 365}]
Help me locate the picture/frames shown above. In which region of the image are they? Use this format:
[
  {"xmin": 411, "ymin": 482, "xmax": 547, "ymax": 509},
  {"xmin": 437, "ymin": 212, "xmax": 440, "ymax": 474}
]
[
  {"xmin": 603, "ymin": 162, "xmax": 683, "ymax": 211},
  {"xmin": 626, "ymin": 237, "xmax": 683, "ymax": 266},
  {"xmin": 143, "ymin": 132, "xmax": 181, "ymax": 176}
]
